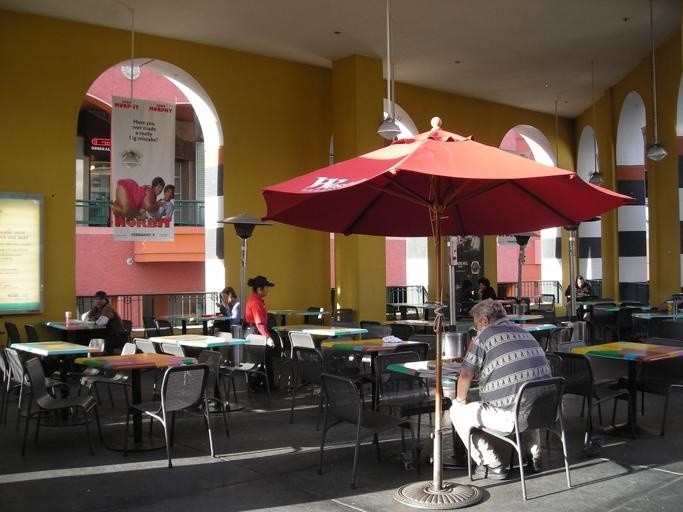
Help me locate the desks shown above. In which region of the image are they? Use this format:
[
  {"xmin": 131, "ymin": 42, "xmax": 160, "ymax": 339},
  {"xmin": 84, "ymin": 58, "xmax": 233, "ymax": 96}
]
[
  {"xmin": 11, "ymin": 341, "xmax": 103, "ymax": 427},
  {"xmin": 320, "ymin": 340, "xmax": 431, "ymax": 411},
  {"xmin": 387, "ymin": 301, "xmax": 573, "ymax": 359},
  {"xmin": 158, "ymin": 314, "xmax": 231, "ymax": 336},
  {"xmin": 295, "ymin": 311, "xmax": 333, "ymax": 325},
  {"xmin": 576, "ymin": 299, "xmax": 681, "ymax": 345},
  {"xmin": 149, "ymin": 334, "xmax": 251, "ymax": 413},
  {"xmin": 271, "ymin": 324, "xmax": 369, "ymax": 389},
  {"xmin": 266, "ymin": 310, "xmax": 295, "ymax": 325},
  {"xmin": 40, "ymin": 319, "xmax": 107, "ymax": 341},
  {"xmin": 72, "ymin": 353, "xmax": 186, "ymax": 453},
  {"xmin": 570, "ymin": 341, "xmax": 683, "ymax": 439}
]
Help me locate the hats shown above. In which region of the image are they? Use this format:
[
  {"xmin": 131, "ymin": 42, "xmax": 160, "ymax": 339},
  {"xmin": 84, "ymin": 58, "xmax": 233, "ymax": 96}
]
[{"xmin": 253, "ymin": 276, "xmax": 273, "ymax": 286}]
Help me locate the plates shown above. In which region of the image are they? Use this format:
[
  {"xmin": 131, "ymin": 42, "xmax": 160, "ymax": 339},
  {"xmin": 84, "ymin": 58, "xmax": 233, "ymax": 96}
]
[{"xmin": 426, "ymin": 359, "xmax": 467, "ymax": 370}]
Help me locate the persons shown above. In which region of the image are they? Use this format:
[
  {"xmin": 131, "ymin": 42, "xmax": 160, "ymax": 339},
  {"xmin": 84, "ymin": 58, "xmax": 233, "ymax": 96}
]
[
  {"xmin": 455, "ymin": 278, "xmax": 473, "ymax": 313},
  {"xmin": 215, "ymin": 292, "xmax": 225, "ymax": 316},
  {"xmin": 221, "ymin": 286, "xmax": 241, "ymax": 331},
  {"xmin": 83, "ymin": 291, "xmax": 121, "ymax": 347},
  {"xmin": 111, "ymin": 176, "xmax": 168, "ymax": 219},
  {"xmin": 244, "ymin": 276, "xmax": 274, "ymax": 393},
  {"xmin": 475, "ymin": 278, "xmax": 497, "ymax": 299},
  {"xmin": 564, "ymin": 275, "xmax": 593, "ymax": 300},
  {"xmin": 449, "ymin": 297, "xmax": 554, "ymax": 481},
  {"xmin": 141, "ymin": 184, "xmax": 174, "ymax": 219}
]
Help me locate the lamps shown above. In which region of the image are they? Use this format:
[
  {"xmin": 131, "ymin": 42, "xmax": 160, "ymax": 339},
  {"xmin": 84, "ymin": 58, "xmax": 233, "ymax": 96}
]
[
  {"xmin": 377, "ymin": 1, "xmax": 400, "ymax": 141},
  {"xmin": 645, "ymin": 1, "xmax": 667, "ymax": 162},
  {"xmin": 119, "ymin": 8, "xmax": 142, "ymax": 169},
  {"xmin": 588, "ymin": 55, "xmax": 604, "ymax": 185}
]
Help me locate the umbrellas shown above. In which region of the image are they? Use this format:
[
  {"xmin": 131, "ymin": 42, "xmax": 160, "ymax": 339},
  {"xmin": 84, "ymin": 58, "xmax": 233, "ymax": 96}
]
[{"xmin": 259, "ymin": 116, "xmax": 638, "ymax": 509}]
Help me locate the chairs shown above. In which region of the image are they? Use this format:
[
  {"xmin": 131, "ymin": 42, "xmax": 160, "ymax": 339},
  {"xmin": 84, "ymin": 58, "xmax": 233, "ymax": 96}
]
[
  {"xmin": 2, "ymin": 348, "xmax": 76, "ymax": 432},
  {"xmin": 318, "ymin": 373, "xmax": 421, "ymax": 489},
  {"xmin": 610, "ymin": 337, "xmax": 681, "ymax": 439},
  {"xmin": 545, "ymin": 352, "xmax": 635, "ymax": 441},
  {"xmin": 150, "ymin": 349, "xmax": 230, "ymax": 444},
  {"xmin": 469, "ymin": 377, "xmax": 571, "ymax": 501},
  {"xmin": 508, "ymin": 352, "xmax": 563, "ymax": 456},
  {"xmin": 373, "ymin": 351, "xmax": 458, "ymax": 468},
  {"xmin": 288, "ymin": 329, "xmax": 371, "ymax": 433},
  {"xmin": 360, "ymin": 295, "xmax": 681, "ymax": 413},
  {"xmin": 122, "ymin": 365, "xmax": 217, "ymax": 468},
  {"xmin": 5, "ymin": 306, "xmax": 353, "ymax": 409},
  {"xmin": 25, "ymin": 356, "xmax": 103, "ymax": 457}
]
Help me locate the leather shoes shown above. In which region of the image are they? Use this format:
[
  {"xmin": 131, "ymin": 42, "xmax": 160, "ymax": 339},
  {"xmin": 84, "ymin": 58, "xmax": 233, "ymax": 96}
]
[
  {"xmin": 474, "ymin": 465, "xmax": 506, "ymax": 479},
  {"xmin": 527, "ymin": 460, "xmax": 543, "ymax": 473}
]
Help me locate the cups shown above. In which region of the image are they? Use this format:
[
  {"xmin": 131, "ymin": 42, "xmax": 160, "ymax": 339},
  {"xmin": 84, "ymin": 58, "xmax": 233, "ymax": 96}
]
[
  {"xmin": 64, "ymin": 323, "xmax": 70, "ymax": 329},
  {"xmin": 65, "ymin": 311, "xmax": 72, "ymax": 322},
  {"xmin": 666, "ymin": 300, "xmax": 674, "ymax": 314}
]
